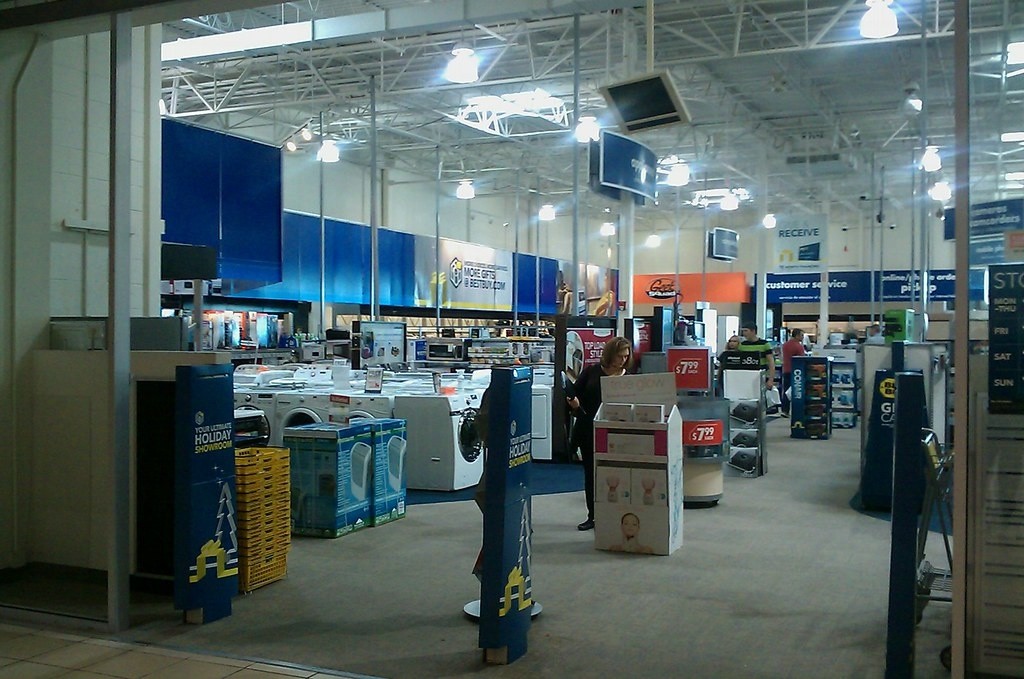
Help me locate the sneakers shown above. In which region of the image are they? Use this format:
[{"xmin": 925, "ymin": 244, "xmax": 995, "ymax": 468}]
[{"xmin": 578, "ymin": 519, "xmax": 595, "ymax": 531}]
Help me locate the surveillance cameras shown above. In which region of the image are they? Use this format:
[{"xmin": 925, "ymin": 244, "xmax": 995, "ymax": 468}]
[
  {"xmin": 890, "ymin": 225, "xmax": 895, "ymax": 229},
  {"xmin": 860, "ymin": 195, "xmax": 867, "ymax": 199},
  {"xmin": 903, "ymin": 82, "xmax": 919, "ymax": 96},
  {"xmin": 842, "ymin": 226, "xmax": 848, "ymax": 231}
]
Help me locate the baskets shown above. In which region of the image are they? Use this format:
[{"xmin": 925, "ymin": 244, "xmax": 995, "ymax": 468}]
[{"xmin": 234, "ymin": 447, "xmax": 291, "ymax": 592}]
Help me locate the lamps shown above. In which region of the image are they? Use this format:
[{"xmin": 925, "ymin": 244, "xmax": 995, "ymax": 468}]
[
  {"xmin": 442, "ymin": 0, "xmax": 1024, "ymax": 246},
  {"xmin": 317, "ymin": 112, "xmax": 340, "ymax": 163}
]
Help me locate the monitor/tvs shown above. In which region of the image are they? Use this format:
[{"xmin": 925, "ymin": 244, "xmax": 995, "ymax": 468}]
[
  {"xmin": 351, "ymin": 320, "xmax": 407, "ymax": 370},
  {"xmin": 652, "ymin": 305, "xmax": 675, "ymax": 354},
  {"xmin": 692, "ymin": 322, "xmax": 706, "ymax": 340},
  {"xmin": 598, "ymin": 69, "xmax": 693, "ymax": 136}
]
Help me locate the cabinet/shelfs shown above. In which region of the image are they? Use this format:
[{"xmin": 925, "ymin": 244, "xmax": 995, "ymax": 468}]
[{"xmin": 812, "ymin": 349, "xmax": 862, "ymax": 427}]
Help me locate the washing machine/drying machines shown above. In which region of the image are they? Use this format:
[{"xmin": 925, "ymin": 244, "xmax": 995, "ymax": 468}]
[{"xmin": 231, "ymin": 362, "xmax": 556, "ymax": 491}]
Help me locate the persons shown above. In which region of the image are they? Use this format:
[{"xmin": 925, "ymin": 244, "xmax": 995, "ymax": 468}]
[
  {"xmin": 567, "ymin": 336, "xmax": 632, "ymax": 531},
  {"xmin": 717, "ymin": 335, "xmax": 740, "ymax": 384},
  {"xmin": 861, "ymin": 324, "xmax": 885, "ymax": 344},
  {"xmin": 781, "ymin": 328, "xmax": 805, "ymax": 417},
  {"xmin": 555, "ymin": 270, "xmax": 572, "ymax": 314},
  {"xmin": 736, "ymin": 322, "xmax": 775, "ymax": 390}
]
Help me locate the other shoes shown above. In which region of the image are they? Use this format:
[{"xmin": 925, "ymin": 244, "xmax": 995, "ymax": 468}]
[{"xmin": 781, "ymin": 412, "xmax": 789, "ymax": 418}]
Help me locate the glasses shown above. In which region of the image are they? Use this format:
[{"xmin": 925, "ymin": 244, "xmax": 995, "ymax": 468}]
[{"xmin": 729, "ymin": 340, "xmax": 738, "ymax": 343}]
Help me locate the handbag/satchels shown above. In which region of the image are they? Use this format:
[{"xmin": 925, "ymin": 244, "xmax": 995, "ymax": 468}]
[{"xmin": 766, "ymin": 386, "xmax": 782, "ymax": 409}]
